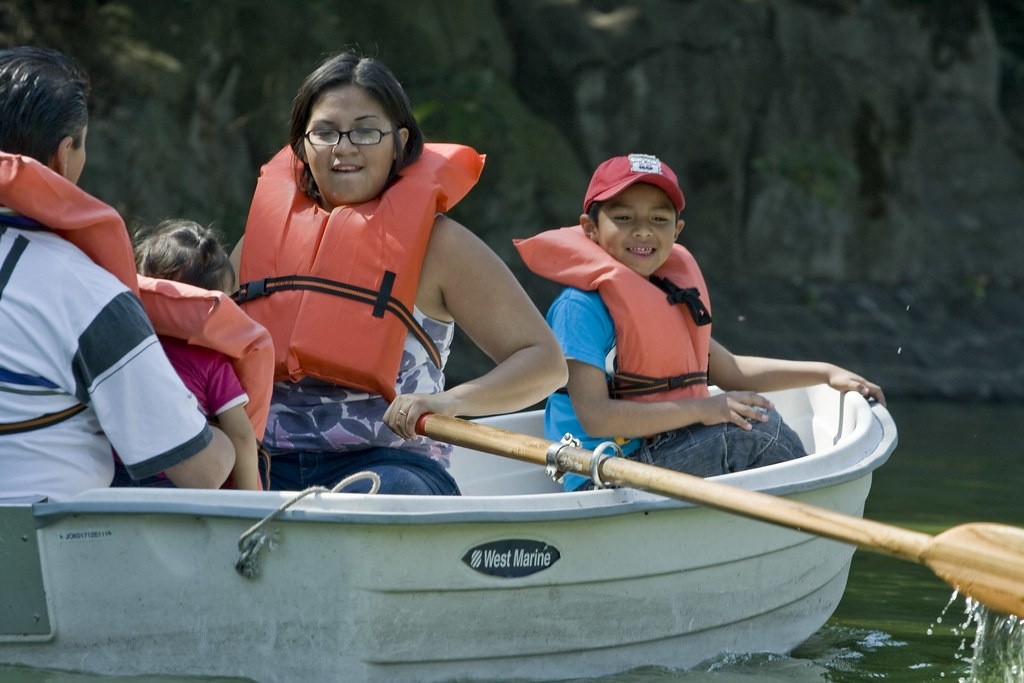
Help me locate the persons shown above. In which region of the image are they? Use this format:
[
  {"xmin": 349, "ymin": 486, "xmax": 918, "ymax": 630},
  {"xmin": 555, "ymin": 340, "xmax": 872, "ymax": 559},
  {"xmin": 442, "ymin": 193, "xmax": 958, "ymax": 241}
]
[
  {"xmin": 222, "ymin": 43, "xmax": 569, "ymax": 496},
  {"xmin": 111, "ymin": 218, "xmax": 258, "ymax": 490},
  {"xmin": 0, "ymin": 49, "xmax": 235, "ymax": 504},
  {"xmin": 544, "ymin": 154, "xmax": 887, "ymax": 493}
]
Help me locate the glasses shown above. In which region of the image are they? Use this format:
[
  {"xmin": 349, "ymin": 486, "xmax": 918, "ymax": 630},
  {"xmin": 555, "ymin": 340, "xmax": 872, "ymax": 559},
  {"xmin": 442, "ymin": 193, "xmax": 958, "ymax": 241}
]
[{"xmin": 302, "ymin": 128, "xmax": 394, "ymax": 146}]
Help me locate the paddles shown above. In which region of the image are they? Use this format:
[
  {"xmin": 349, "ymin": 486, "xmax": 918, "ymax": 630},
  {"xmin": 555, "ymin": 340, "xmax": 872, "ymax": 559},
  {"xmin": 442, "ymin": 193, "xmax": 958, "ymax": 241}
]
[{"xmin": 413, "ymin": 413, "xmax": 1023, "ymax": 618}]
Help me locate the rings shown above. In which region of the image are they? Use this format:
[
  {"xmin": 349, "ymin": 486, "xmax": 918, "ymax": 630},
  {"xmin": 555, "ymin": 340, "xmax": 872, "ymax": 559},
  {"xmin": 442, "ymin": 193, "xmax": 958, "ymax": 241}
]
[{"xmin": 398, "ymin": 410, "xmax": 407, "ymax": 416}]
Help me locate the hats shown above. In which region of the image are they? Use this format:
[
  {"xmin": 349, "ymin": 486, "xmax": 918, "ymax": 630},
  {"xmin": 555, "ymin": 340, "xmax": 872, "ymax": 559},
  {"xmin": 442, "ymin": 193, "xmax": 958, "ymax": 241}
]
[{"xmin": 583, "ymin": 153, "xmax": 685, "ymax": 218}]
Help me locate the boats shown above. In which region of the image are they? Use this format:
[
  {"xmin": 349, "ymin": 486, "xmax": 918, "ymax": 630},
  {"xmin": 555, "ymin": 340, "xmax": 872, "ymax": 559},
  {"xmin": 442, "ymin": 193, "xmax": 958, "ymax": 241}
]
[{"xmin": 0, "ymin": 367, "xmax": 901, "ymax": 682}]
